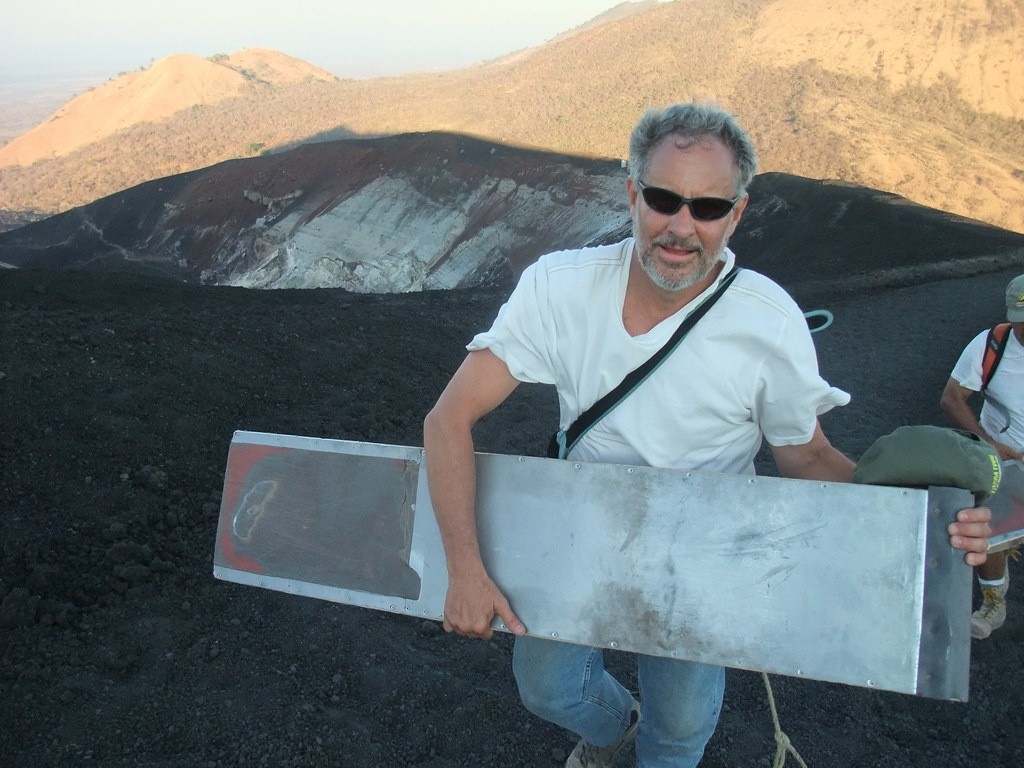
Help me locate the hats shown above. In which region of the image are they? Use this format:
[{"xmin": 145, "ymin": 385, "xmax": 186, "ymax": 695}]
[
  {"xmin": 852, "ymin": 427, "xmax": 1002, "ymax": 509},
  {"xmin": 1005, "ymin": 274, "xmax": 1024, "ymax": 323}
]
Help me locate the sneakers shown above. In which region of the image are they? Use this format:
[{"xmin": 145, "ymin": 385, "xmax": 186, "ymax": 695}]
[
  {"xmin": 563, "ymin": 696, "xmax": 642, "ymax": 768},
  {"xmin": 970, "ymin": 568, "xmax": 1010, "ymax": 639}
]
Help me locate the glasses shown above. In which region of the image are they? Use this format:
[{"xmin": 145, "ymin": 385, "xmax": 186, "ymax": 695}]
[{"xmin": 629, "ymin": 172, "xmax": 740, "ymax": 221}]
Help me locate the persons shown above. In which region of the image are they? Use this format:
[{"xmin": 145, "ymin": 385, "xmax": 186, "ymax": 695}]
[
  {"xmin": 422, "ymin": 103, "xmax": 991, "ymax": 767},
  {"xmin": 941, "ymin": 265, "xmax": 1024, "ymax": 638}
]
